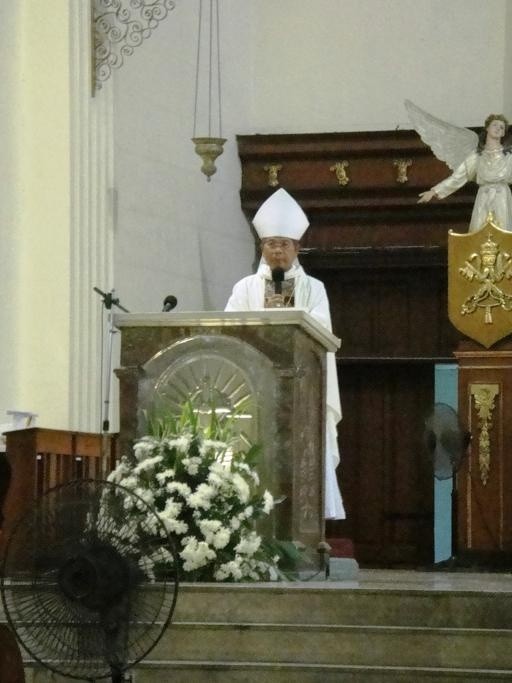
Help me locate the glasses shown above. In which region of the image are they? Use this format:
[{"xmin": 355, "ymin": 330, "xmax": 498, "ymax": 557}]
[{"xmin": 263, "ymin": 239, "xmax": 296, "ymax": 249}]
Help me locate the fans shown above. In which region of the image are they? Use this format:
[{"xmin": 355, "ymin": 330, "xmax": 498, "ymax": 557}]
[
  {"xmin": 0, "ymin": 478, "xmax": 180, "ymax": 682},
  {"xmin": 411, "ymin": 402, "xmax": 493, "ymax": 574}
]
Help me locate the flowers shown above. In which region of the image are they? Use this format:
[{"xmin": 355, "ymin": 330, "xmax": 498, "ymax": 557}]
[{"xmin": 82, "ymin": 388, "xmax": 315, "ymax": 583}]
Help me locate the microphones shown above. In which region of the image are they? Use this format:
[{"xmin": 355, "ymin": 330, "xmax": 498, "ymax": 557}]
[
  {"xmin": 271, "ymin": 266, "xmax": 285, "ymax": 295},
  {"xmin": 158, "ymin": 295, "xmax": 178, "ymax": 311}
]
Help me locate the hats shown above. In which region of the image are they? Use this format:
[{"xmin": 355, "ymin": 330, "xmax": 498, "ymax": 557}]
[{"xmin": 251, "ymin": 186, "xmax": 310, "ymax": 240}]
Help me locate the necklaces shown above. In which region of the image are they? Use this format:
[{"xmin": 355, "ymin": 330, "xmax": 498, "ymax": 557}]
[{"xmin": 284, "ymin": 276, "xmax": 299, "ymax": 308}]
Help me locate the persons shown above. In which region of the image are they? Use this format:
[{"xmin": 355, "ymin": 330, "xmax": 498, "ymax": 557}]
[
  {"xmin": 224, "ymin": 184, "xmax": 350, "ymax": 521},
  {"xmin": 416, "ymin": 113, "xmax": 512, "ymax": 234}
]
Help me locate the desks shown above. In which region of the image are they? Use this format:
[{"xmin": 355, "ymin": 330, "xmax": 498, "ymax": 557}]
[{"xmin": 0, "ymin": 427, "xmax": 121, "ymax": 577}]
